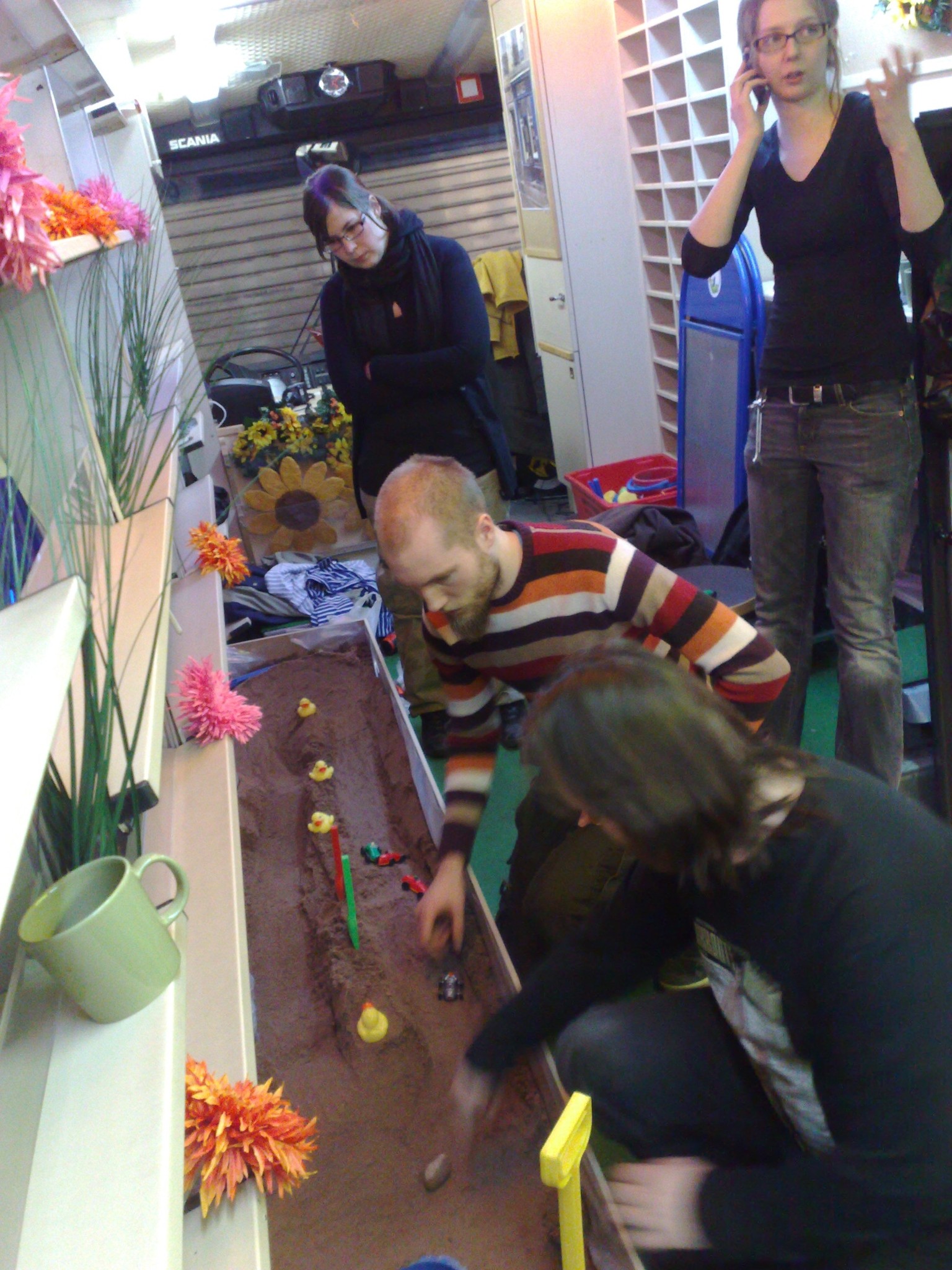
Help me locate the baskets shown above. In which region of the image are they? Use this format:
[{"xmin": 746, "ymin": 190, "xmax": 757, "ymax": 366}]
[{"xmin": 564, "ymin": 452, "xmax": 679, "ymax": 521}]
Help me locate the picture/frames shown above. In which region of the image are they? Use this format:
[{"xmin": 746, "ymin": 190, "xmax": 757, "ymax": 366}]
[{"xmin": 488, "ymin": 0, "xmax": 560, "ymax": 260}]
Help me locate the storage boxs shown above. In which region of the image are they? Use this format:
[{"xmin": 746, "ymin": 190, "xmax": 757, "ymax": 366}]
[{"xmin": 564, "ymin": 453, "xmax": 677, "ymax": 516}]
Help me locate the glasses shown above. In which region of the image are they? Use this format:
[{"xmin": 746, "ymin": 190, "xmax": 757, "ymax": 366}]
[
  {"xmin": 753, "ymin": 18, "xmax": 830, "ymax": 53},
  {"xmin": 318, "ymin": 201, "xmax": 370, "ymax": 254}
]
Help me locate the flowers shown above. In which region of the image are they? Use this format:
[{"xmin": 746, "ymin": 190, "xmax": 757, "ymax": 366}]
[
  {"xmin": 234, "ymin": 391, "xmax": 374, "ymax": 553},
  {"xmin": 187, "ymin": 520, "xmax": 250, "ymax": 588},
  {"xmin": 168, "ymin": 657, "xmax": 263, "ymax": 746},
  {"xmin": 0, "ymin": 70, "xmax": 149, "ymax": 291}
]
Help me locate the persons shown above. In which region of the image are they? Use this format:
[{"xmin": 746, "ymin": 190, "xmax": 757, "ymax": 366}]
[
  {"xmin": 683, "ymin": 0, "xmax": 944, "ymax": 792},
  {"xmin": 900, "ymin": 107, "xmax": 952, "ymax": 822},
  {"xmin": 454, "ymin": 643, "xmax": 952, "ymax": 1270},
  {"xmin": 303, "ymin": 167, "xmax": 521, "ymax": 763},
  {"xmin": 374, "ymin": 453, "xmax": 792, "ymax": 959}
]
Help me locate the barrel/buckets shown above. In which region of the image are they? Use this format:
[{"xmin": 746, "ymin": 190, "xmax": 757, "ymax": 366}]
[{"xmin": 626, "ymin": 466, "xmax": 678, "ymax": 498}]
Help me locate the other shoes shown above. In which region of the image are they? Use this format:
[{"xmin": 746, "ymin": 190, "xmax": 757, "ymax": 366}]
[{"xmin": 652, "ymin": 954, "xmax": 708, "ymax": 989}]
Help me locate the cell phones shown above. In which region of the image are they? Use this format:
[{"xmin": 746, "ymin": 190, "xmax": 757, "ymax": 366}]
[{"xmin": 743, "ymin": 53, "xmax": 768, "ymax": 104}]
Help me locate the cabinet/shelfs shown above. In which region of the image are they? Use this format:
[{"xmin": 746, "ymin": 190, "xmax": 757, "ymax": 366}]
[
  {"xmin": 612, "ymin": 0, "xmax": 773, "ymax": 455},
  {"xmin": 0, "ymin": 0, "xmax": 270, "ymax": 1270}
]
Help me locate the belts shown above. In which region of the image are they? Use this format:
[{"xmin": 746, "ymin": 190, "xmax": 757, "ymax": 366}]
[{"xmin": 760, "ymin": 374, "xmax": 903, "ymax": 406}]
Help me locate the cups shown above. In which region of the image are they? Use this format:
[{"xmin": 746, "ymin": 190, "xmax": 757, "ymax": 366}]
[{"xmin": 17, "ymin": 852, "xmax": 191, "ymax": 1022}]
